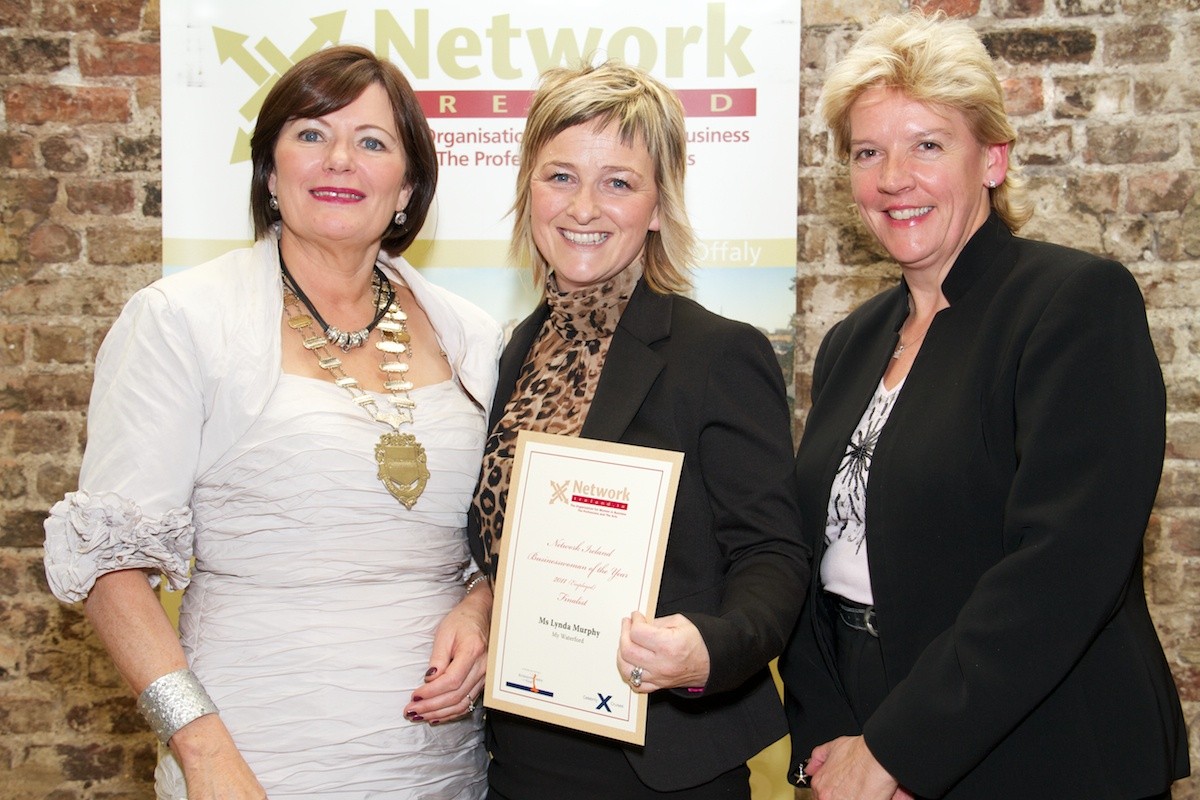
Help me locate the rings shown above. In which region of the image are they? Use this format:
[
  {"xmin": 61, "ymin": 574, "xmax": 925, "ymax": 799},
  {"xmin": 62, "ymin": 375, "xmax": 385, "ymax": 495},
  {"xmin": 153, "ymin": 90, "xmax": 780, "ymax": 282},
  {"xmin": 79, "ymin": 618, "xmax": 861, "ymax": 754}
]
[
  {"xmin": 629, "ymin": 668, "xmax": 643, "ymax": 687},
  {"xmin": 467, "ymin": 695, "xmax": 475, "ymax": 712}
]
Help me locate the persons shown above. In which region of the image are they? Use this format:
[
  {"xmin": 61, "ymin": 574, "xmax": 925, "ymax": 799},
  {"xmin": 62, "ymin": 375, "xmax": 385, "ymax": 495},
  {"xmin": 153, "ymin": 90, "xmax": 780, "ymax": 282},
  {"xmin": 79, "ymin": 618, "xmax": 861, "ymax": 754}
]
[
  {"xmin": 464, "ymin": 62, "xmax": 810, "ymax": 800},
  {"xmin": 778, "ymin": 14, "xmax": 1191, "ymax": 800},
  {"xmin": 41, "ymin": 46, "xmax": 507, "ymax": 800}
]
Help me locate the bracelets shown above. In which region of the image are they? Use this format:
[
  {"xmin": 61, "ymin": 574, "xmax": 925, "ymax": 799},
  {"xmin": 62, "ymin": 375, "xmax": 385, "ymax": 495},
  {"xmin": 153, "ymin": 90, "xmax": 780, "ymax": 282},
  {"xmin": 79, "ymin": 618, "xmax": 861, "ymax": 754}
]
[
  {"xmin": 137, "ymin": 670, "xmax": 216, "ymax": 747},
  {"xmin": 467, "ymin": 575, "xmax": 487, "ymax": 594}
]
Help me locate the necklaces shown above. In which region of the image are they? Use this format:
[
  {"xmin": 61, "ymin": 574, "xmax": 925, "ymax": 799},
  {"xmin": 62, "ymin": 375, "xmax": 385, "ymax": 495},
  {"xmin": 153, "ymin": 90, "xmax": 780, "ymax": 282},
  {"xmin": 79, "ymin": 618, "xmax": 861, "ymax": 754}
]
[
  {"xmin": 892, "ymin": 326, "xmax": 926, "ymax": 360},
  {"xmin": 279, "ymin": 250, "xmax": 431, "ymax": 509}
]
